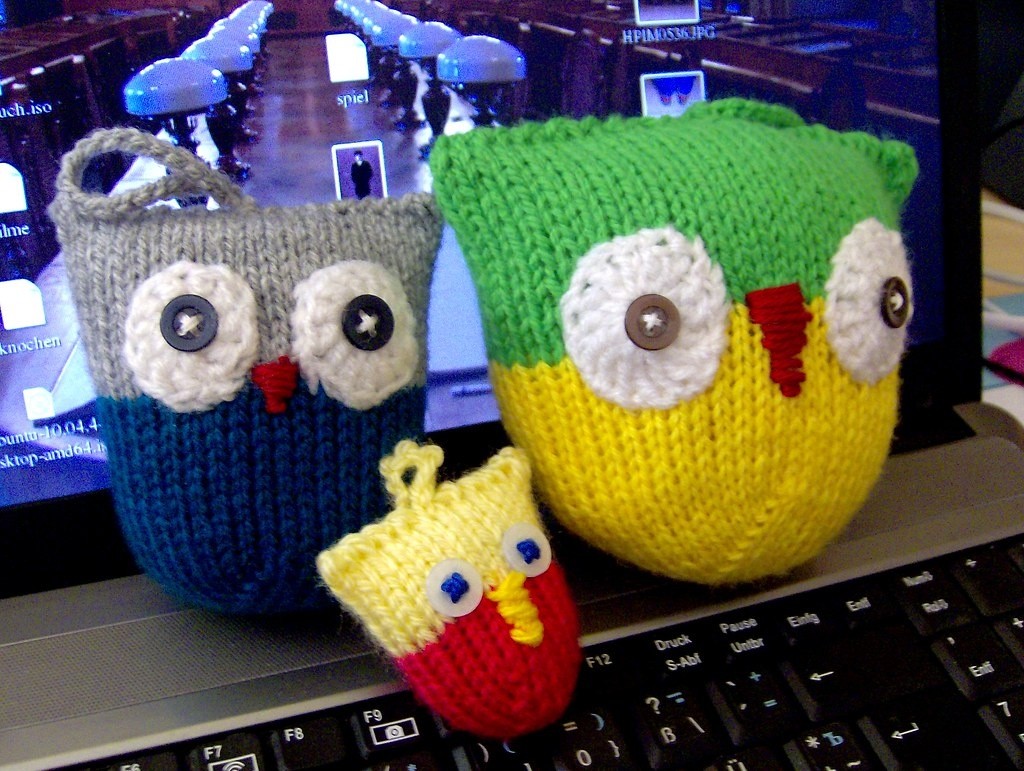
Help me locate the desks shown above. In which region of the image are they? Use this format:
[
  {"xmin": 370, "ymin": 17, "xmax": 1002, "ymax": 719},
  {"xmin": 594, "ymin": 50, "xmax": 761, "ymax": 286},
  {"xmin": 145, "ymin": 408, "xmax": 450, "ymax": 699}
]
[{"xmin": 1, "ymin": 29, "xmax": 497, "ymax": 502}]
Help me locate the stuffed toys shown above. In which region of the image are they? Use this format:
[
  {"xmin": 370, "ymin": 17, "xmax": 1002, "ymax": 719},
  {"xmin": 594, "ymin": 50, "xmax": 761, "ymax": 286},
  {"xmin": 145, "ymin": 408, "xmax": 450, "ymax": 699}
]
[
  {"xmin": 431, "ymin": 98, "xmax": 916, "ymax": 582},
  {"xmin": 314, "ymin": 439, "xmax": 579, "ymax": 734},
  {"xmin": 53, "ymin": 127, "xmax": 444, "ymax": 621}
]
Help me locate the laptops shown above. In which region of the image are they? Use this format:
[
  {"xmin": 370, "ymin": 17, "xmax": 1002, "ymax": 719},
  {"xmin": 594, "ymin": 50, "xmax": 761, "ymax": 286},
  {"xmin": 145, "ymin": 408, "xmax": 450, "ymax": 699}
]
[{"xmin": 0, "ymin": 0, "xmax": 1024, "ymax": 771}]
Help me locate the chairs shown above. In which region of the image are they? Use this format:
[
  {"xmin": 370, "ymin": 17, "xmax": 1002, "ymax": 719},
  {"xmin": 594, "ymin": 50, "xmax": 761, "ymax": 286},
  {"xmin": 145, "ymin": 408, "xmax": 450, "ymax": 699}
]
[{"xmin": 0, "ymin": 1, "xmax": 948, "ymax": 291}]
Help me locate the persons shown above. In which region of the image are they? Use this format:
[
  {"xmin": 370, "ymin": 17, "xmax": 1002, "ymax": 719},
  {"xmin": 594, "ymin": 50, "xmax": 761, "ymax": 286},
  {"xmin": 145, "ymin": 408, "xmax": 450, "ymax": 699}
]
[
  {"xmin": 651, "ymin": 77, "xmax": 696, "ymax": 106},
  {"xmin": 351, "ymin": 150, "xmax": 374, "ymax": 198}
]
